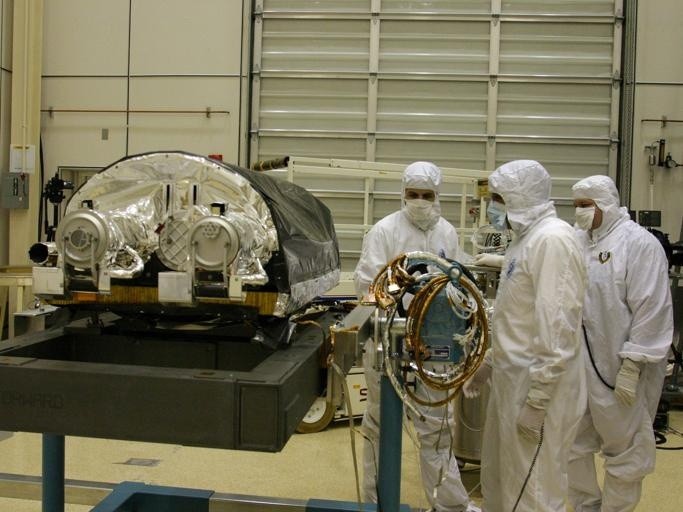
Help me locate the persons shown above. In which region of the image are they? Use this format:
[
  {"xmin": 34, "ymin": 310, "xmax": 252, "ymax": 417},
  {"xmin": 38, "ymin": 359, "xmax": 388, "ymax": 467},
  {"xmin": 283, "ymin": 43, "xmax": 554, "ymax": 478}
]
[
  {"xmin": 352, "ymin": 159, "xmax": 481, "ymax": 512},
  {"xmin": 565, "ymin": 174, "xmax": 673, "ymax": 512},
  {"xmin": 480, "ymin": 156, "xmax": 588, "ymax": 512}
]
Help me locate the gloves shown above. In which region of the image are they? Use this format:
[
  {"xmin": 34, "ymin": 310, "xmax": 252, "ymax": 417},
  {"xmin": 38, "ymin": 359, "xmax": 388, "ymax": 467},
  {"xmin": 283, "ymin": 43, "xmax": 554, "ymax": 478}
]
[
  {"xmin": 474, "ymin": 252, "xmax": 505, "ymax": 269},
  {"xmin": 515, "ymin": 387, "xmax": 552, "ymax": 445},
  {"xmin": 613, "ymin": 357, "xmax": 642, "ymax": 410},
  {"xmin": 462, "ymin": 363, "xmax": 493, "ymax": 400}
]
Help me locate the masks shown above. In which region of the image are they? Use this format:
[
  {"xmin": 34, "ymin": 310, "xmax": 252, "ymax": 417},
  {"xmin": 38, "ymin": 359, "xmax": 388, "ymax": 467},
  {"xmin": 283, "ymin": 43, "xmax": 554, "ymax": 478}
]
[
  {"xmin": 575, "ymin": 205, "xmax": 597, "ymax": 231},
  {"xmin": 486, "ymin": 199, "xmax": 509, "ymax": 231},
  {"xmin": 404, "ymin": 198, "xmax": 435, "ymax": 222}
]
